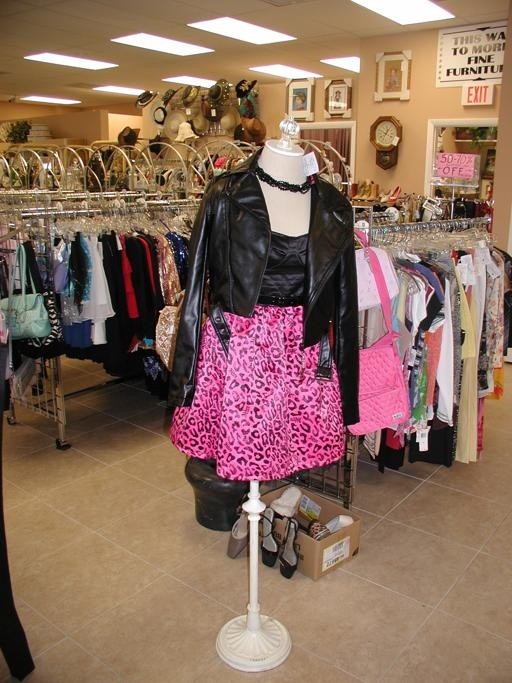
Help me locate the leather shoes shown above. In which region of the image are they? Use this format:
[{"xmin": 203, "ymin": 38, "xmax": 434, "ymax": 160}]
[
  {"xmin": 352, "ymin": 179, "xmax": 401, "ymax": 202},
  {"xmin": 228, "ymin": 486, "xmax": 354, "ymax": 579}
]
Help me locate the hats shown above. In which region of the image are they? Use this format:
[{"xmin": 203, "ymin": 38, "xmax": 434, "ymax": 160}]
[
  {"xmin": 135, "ymin": 79, "xmax": 268, "ymax": 159},
  {"xmin": 117, "ymin": 127, "xmax": 139, "ymax": 144}
]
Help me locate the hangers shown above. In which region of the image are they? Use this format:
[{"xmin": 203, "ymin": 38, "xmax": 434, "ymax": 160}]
[{"xmin": 369, "ymin": 214, "xmax": 499, "ymax": 268}]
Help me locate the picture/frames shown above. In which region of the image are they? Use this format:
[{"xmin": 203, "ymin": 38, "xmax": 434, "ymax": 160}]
[
  {"xmin": 284, "ymin": 75, "xmax": 353, "ymax": 121},
  {"xmin": 373, "ymin": 48, "xmax": 413, "ymax": 101}
]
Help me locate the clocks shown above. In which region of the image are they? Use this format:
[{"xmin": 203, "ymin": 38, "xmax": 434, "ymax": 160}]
[{"xmin": 369, "ymin": 115, "xmax": 402, "ymax": 169}]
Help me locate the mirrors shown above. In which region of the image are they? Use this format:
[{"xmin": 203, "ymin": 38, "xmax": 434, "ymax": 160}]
[{"xmin": 424, "ymin": 117, "xmax": 498, "ymax": 232}]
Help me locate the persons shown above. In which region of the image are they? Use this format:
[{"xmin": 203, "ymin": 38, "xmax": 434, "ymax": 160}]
[
  {"xmin": 294, "ymin": 93, "xmax": 306, "ymax": 113},
  {"xmin": 170, "ymin": 140, "xmax": 359, "ymax": 480}
]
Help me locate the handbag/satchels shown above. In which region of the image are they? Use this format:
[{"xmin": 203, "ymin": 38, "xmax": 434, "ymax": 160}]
[
  {"xmin": 11, "ymin": 358, "xmax": 36, "ymax": 398},
  {"xmin": 1, "ymin": 288, "xmax": 64, "ymax": 358},
  {"xmin": 348, "ymin": 334, "xmax": 412, "ymax": 436}
]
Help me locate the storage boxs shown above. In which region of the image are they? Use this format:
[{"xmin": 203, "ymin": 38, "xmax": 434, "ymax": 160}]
[{"xmin": 257, "ymin": 483, "xmax": 361, "ymax": 582}]
